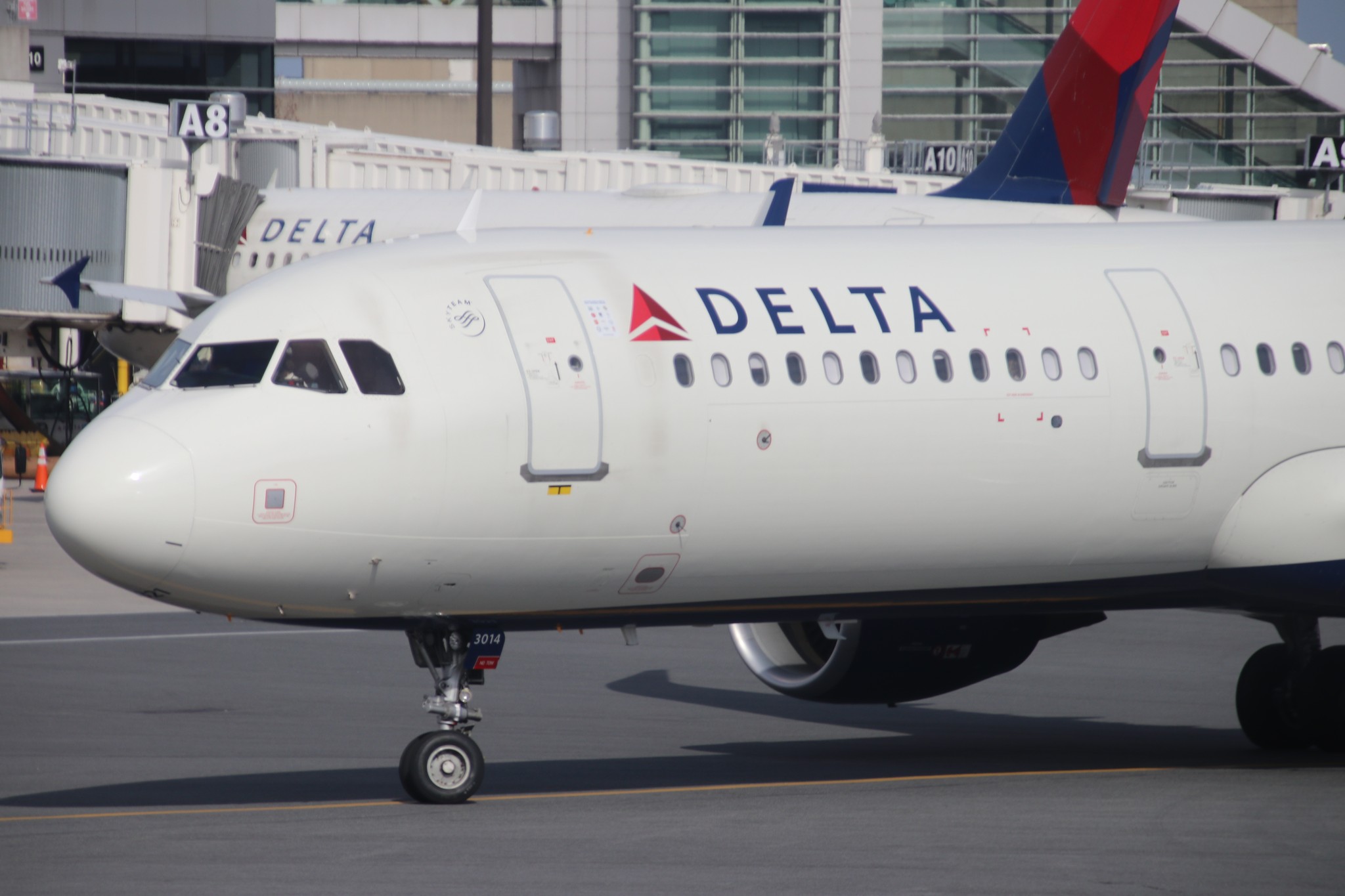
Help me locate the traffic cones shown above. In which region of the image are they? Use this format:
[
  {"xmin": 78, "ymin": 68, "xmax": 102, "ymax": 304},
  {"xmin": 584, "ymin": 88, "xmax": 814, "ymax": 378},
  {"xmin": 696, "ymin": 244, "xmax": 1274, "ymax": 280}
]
[{"xmin": 28, "ymin": 442, "xmax": 50, "ymax": 494}]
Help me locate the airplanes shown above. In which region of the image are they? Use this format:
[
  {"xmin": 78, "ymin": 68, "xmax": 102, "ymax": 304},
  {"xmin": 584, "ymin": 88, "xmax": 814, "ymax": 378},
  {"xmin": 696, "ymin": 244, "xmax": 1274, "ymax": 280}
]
[{"xmin": 42, "ymin": 1, "xmax": 1345, "ymax": 802}]
